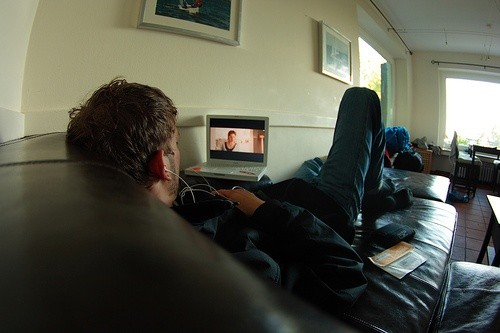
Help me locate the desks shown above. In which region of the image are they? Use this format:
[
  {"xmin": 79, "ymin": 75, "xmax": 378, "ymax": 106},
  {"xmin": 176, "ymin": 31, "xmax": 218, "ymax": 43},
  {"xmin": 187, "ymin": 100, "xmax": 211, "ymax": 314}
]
[{"xmin": 467, "ymin": 143, "xmax": 500, "ymax": 197}]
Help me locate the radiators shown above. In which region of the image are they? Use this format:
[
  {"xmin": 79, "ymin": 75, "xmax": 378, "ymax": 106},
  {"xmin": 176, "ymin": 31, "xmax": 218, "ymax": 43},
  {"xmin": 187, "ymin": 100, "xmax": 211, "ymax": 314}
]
[{"xmin": 453, "ymin": 160, "xmax": 500, "ymax": 184}]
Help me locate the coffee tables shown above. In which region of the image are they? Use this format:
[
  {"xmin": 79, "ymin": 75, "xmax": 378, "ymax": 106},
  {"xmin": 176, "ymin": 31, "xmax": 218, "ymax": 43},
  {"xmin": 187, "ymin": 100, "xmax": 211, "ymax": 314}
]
[{"xmin": 474, "ymin": 194, "xmax": 500, "ymax": 268}]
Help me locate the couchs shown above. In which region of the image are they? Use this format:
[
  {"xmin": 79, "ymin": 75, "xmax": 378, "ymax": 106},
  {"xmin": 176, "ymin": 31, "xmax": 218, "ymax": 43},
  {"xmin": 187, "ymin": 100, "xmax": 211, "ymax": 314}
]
[{"xmin": 1, "ymin": 132, "xmax": 500, "ymax": 333}]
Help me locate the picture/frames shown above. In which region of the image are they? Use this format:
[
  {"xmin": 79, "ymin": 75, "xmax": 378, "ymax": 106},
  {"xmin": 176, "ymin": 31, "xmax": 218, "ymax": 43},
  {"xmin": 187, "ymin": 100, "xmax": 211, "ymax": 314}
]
[
  {"xmin": 318, "ymin": 20, "xmax": 353, "ymax": 86},
  {"xmin": 136, "ymin": 0, "xmax": 243, "ymax": 46}
]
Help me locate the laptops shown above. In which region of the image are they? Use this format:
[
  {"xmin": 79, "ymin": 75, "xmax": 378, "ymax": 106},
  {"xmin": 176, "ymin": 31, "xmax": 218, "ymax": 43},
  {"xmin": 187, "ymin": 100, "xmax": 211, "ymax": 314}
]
[{"xmin": 185, "ymin": 115, "xmax": 270, "ymax": 181}]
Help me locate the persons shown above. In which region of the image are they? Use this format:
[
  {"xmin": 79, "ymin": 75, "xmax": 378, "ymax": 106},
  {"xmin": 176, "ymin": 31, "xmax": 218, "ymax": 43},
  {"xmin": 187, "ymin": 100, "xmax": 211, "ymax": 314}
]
[
  {"xmin": 64, "ymin": 75, "xmax": 415, "ymax": 313},
  {"xmin": 219, "ymin": 130, "xmax": 241, "ymax": 152}
]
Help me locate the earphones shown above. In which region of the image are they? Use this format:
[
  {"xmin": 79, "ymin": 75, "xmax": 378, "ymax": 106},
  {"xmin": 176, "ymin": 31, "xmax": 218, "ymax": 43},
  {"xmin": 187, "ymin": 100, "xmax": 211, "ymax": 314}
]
[{"xmin": 164, "ymin": 166, "xmax": 167, "ymax": 172}]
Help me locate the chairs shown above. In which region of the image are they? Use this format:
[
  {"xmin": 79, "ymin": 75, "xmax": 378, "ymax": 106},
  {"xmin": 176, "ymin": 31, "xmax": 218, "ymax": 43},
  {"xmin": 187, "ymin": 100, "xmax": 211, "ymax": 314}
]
[{"xmin": 449, "ymin": 130, "xmax": 481, "ymax": 199}]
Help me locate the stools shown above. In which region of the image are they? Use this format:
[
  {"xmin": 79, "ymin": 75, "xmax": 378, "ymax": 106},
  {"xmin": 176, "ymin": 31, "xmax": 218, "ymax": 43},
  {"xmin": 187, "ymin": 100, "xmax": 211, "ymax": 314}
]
[{"xmin": 381, "ymin": 166, "xmax": 452, "ymax": 201}]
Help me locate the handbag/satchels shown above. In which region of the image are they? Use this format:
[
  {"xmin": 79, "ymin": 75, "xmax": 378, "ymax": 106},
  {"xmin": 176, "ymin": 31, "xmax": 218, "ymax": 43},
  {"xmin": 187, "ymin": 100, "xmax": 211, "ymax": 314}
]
[
  {"xmin": 386, "ymin": 126, "xmax": 410, "ymax": 148},
  {"xmin": 393, "ymin": 151, "xmax": 424, "ymax": 172}
]
